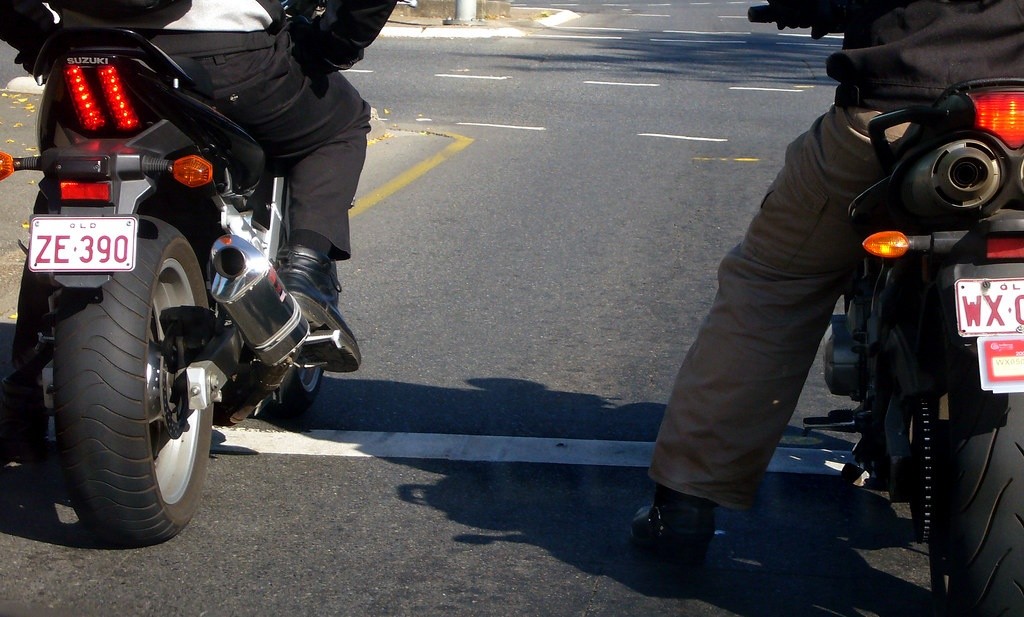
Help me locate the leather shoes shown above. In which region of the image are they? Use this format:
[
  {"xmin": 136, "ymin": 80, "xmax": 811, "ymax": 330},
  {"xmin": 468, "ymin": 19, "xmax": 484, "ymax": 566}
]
[
  {"xmin": 2, "ymin": 369, "xmax": 39, "ymax": 390},
  {"xmin": 274, "ymin": 242, "xmax": 361, "ymax": 373},
  {"xmin": 630, "ymin": 505, "xmax": 715, "ymax": 545}
]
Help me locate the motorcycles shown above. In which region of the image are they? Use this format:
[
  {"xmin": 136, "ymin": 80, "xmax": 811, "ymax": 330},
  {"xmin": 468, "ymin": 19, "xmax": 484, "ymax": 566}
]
[
  {"xmin": 749, "ymin": 1, "xmax": 1023, "ymax": 617},
  {"xmin": 2, "ymin": 0, "xmax": 343, "ymax": 548}
]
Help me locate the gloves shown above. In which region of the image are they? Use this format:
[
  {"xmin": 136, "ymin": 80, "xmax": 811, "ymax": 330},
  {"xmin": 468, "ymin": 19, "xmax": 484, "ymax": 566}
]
[{"xmin": 768, "ymin": 0, "xmax": 814, "ymax": 30}]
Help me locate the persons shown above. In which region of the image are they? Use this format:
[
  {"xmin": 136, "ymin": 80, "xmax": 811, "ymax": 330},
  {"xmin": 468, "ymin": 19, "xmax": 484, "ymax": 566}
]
[
  {"xmin": 0, "ymin": 0, "xmax": 397, "ymax": 452},
  {"xmin": 630, "ymin": 0, "xmax": 1024, "ymax": 575}
]
